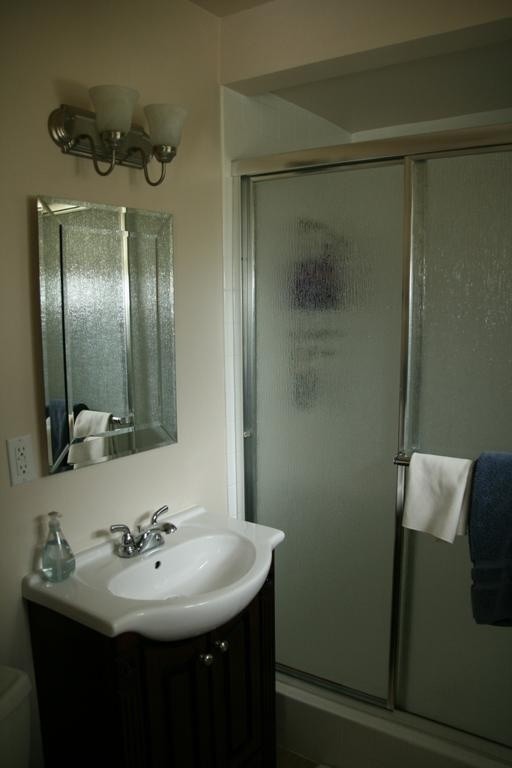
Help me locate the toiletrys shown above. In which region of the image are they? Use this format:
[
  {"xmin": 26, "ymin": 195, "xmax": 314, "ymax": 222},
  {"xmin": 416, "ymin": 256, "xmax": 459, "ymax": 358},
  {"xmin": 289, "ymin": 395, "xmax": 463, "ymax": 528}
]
[{"xmin": 42, "ymin": 512, "xmax": 75, "ymax": 581}]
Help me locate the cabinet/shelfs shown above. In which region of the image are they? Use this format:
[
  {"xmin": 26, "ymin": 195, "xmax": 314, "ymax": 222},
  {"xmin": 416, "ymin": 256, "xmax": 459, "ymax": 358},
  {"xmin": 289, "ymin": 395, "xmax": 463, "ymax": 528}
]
[{"xmin": 24, "ymin": 549, "xmax": 276, "ymax": 768}]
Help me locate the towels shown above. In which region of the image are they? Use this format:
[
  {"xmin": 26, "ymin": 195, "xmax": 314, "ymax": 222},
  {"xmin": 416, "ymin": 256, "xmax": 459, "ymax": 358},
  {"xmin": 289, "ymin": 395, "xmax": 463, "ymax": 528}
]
[
  {"xmin": 401, "ymin": 452, "xmax": 473, "ymax": 544},
  {"xmin": 48, "ymin": 399, "xmax": 69, "ymax": 467},
  {"xmin": 469, "ymin": 452, "xmax": 512, "ymax": 627},
  {"xmin": 68, "ymin": 410, "xmax": 113, "ymax": 462}
]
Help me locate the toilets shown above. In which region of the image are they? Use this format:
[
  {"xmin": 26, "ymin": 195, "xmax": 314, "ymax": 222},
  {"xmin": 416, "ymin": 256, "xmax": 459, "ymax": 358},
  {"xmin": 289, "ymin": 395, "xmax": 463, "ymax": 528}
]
[{"xmin": 0, "ymin": 664, "xmax": 31, "ymax": 768}]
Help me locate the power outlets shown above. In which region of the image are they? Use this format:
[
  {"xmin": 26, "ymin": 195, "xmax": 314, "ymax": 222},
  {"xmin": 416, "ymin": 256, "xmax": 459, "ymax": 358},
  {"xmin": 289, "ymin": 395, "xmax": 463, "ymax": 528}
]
[{"xmin": 6, "ymin": 434, "xmax": 38, "ymax": 488}]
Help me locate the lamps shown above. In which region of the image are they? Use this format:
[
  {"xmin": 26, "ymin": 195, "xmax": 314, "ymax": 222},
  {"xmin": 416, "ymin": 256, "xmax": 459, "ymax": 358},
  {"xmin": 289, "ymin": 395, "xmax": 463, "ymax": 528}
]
[{"xmin": 47, "ymin": 84, "xmax": 187, "ymax": 187}]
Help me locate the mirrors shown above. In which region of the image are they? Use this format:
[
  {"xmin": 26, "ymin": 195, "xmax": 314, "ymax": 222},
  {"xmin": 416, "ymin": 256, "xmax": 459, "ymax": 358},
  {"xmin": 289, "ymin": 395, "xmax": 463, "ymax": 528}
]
[{"xmin": 36, "ymin": 194, "xmax": 177, "ymax": 476}]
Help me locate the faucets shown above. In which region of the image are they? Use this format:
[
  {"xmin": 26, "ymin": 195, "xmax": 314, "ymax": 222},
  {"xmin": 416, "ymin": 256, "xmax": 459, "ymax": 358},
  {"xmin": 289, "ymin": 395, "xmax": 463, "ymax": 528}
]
[{"xmin": 135, "ymin": 522, "xmax": 177, "ymax": 552}]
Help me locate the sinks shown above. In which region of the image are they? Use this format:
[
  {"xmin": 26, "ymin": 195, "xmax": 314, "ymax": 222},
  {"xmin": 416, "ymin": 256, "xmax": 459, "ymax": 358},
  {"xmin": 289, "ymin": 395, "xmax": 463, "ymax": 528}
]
[{"xmin": 22, "ymin": 506, "xmax": 286, "ymax": 640}]
